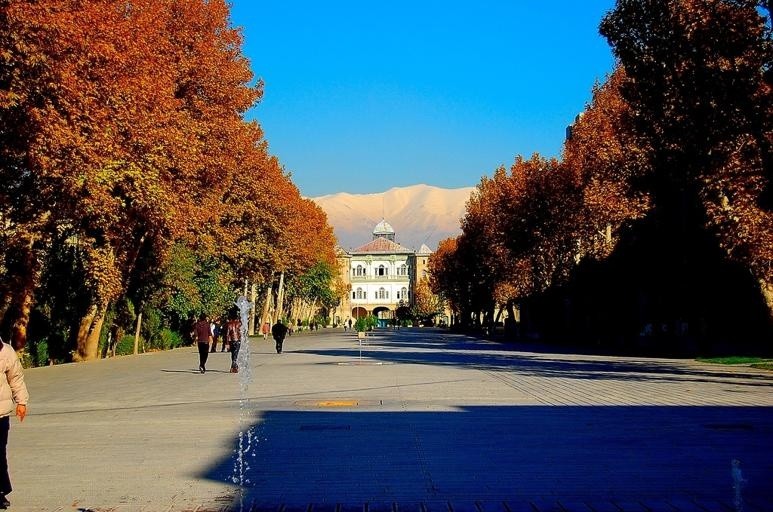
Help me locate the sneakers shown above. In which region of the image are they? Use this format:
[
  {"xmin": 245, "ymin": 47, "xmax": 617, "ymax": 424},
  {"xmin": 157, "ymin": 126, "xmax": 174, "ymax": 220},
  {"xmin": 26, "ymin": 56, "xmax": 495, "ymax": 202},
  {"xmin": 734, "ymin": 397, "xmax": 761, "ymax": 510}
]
[
  {"xmin": 199, "ymin": 366, "xmax": 205, "ymax": 374},
  {"xmin": 230, "ymin": 367, "xmax": 238, "ymax": 373},
  {"xmin": 209, "ymin": 349, "xmax": 230, "ymax": 353}
]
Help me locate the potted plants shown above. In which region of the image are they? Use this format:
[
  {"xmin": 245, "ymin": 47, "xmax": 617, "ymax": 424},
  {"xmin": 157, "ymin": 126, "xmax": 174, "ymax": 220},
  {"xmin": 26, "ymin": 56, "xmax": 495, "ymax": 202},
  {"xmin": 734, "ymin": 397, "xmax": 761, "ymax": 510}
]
[{"xmin": 353, "ymin": 315, "xmax": 378, "ymax": 338}]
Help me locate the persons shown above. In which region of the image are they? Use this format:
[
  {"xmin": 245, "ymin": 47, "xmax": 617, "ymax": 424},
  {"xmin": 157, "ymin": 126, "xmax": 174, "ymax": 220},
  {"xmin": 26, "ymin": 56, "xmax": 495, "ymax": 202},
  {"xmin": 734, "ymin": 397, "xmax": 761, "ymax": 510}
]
[
  {"xmin": 287, "ymin": 316, "xmax": 352, "ymax": 336},
  {"xmin": 272, "ymin": 319, "xmax": 287, "ymax": 353},
  {"xmin": 187, "ymin": 312, "xmax": 241, "ymax": 375},
  {"xmin": 262, "ymin": 321, "xmax": 269, "ymax": 341},
  {"xmin": 0, "ymin": 338, "xmax": 30, "ymax": 511}
]
